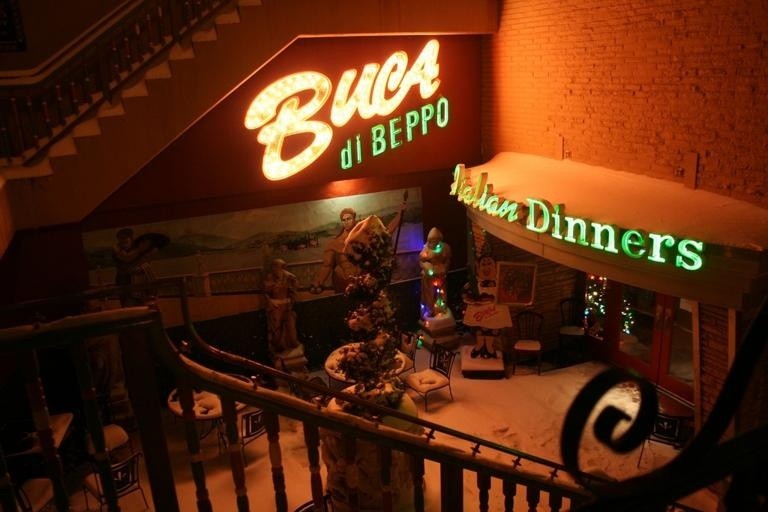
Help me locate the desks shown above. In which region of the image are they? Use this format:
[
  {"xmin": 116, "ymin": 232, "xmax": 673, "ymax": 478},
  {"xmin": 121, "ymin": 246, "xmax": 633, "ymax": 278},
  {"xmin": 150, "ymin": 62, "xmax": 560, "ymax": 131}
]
[
  {"xmin": 165, "ymin": 374, "xmax": 259, "ymax": 453},
  {"xmin": 322, "ymin": 343, "xmax": 405, "ymax": 391}
]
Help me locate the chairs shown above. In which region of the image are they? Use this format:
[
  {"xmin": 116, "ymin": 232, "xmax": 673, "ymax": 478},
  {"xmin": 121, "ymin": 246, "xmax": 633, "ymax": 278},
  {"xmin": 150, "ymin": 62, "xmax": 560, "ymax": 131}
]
[
  {"xmin": 556, "ymin": 297, "xmax": 586, "ymax": 365},
  {"xmin": 217, "ymin": 406, "xmax": 267, "ymax": 467},
  {"xmin": 633, "ymin": 413, "xmax": 692, "ymax": 471},
  {"xmin": 398, "ymin": 330, "xmax": 416, "ymax": 375},
  {"xmin": 403, "ymin": 341, "xmax": 460, "ymax": 409},
  {"xmin": 512, "ymin": 311, "xmax": 545, "ymax": 375},
  {"xmin": 0, "ymin": 470, "xmax": 56, "ymax": 511},
  {"xmin": 81, "ymin": 392, "xmax": 133, "ymax": 457},
  {"xmin": 83, "ymin": 457, "xmax": 148, "ymax": 512}
]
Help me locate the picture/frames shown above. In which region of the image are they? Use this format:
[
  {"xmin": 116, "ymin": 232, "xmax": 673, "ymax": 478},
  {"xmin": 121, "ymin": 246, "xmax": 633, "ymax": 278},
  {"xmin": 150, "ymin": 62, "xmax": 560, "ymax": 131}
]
[{"xmin": 493, "ymin": 261, "xmax": 538, "ymax": 306}]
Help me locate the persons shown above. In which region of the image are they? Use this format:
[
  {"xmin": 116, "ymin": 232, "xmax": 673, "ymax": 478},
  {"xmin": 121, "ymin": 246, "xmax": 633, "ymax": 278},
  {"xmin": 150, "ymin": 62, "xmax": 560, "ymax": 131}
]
[
  {"xmin": 306, "ymin": 203, "xmax": 410, "ymax": 292},
  {"xmin": 419, "ymin": 225, "xmax": 455, "ymax": 311},
  {"xmin": 256, "ymin": 257, "xmax": 303, "ymax": 354},
  {"xmin": 97, "ymin": 227, "xmax": 153, "ymax": 306},
  {"xmin": 462, "ymin": 255, "xmax": 514, "ymax": 360}
]
